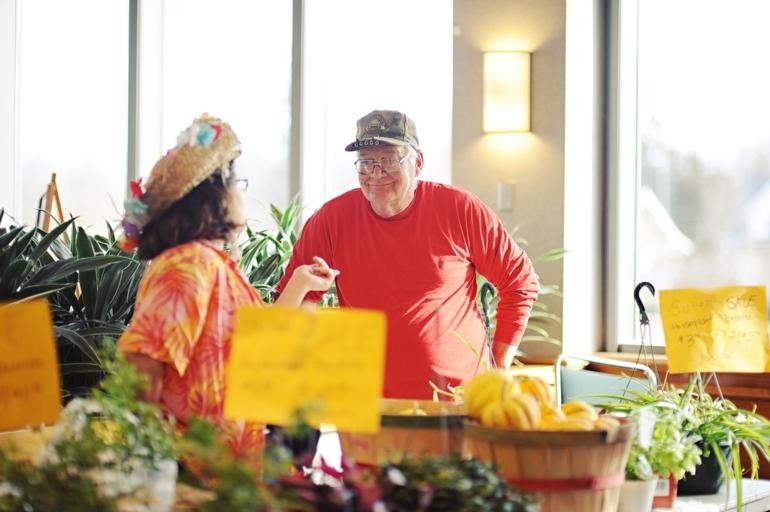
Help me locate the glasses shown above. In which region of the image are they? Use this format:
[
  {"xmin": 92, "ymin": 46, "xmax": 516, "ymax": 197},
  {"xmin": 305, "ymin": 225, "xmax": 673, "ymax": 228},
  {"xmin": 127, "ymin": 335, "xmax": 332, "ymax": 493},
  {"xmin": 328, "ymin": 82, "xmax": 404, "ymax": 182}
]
[
  {"xmin": 230, "ymin": 178, "xmax": 248, "ymax": 193},
  {"xmin": 354, "ymin": 153, "xmax": 411, "ymax": 175}
]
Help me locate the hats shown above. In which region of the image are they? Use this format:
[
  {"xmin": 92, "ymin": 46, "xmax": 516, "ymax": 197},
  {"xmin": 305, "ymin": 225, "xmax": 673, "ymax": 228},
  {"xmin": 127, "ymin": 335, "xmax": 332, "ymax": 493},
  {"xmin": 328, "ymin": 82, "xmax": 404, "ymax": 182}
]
[
  {"xmin": 344, "ymin": 109, "xmax": 420, "ymax": 152},
  {"xmin": 125, "ymin": 119, "xmax": 242, "ymax": 229}
]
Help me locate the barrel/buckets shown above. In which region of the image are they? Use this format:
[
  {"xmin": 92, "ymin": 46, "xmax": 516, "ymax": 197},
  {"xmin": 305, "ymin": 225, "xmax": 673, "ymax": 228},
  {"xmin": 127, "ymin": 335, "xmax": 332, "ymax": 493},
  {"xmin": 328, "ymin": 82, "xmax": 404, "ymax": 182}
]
[
  {"xmin": 456, "ymin": 415, "xmax": 635, "ymax": 512},
  {"xmin": 336, "ymin": 398, "xmax": 475, "ymax": 463}
]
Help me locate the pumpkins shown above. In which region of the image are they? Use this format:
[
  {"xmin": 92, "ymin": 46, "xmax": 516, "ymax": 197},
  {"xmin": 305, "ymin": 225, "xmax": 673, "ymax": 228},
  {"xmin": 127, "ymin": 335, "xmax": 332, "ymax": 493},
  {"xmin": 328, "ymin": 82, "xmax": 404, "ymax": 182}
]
[{"xmin": 464, "ymin": 370, "xmax": 619, "ymax": 431}]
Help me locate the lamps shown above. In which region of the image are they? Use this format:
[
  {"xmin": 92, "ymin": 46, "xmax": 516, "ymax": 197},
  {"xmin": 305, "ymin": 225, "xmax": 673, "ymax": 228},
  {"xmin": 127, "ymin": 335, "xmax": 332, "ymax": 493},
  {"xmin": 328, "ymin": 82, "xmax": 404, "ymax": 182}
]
[{"xmin": 483, "ymin": 50, "xmax": 531, "ymax": 133}]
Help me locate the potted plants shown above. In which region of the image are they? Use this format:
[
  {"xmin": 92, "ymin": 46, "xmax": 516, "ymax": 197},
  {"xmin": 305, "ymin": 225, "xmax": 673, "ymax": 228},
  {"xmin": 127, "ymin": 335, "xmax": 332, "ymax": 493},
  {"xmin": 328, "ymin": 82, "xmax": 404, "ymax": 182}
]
[
  {"xmin": 589, "ymin": 387, "xmax": 769, "ymax": 512},
  {"xmin": 618, "ymin": 394, "xmax": 700, "ymax": 508}
]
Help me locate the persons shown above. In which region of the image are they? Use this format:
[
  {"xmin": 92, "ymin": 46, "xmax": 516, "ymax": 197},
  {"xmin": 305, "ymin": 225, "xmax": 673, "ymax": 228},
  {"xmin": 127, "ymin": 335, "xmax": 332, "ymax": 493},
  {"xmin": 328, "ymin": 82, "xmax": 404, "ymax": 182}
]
[
  {"xmin": 115, "ymin": 113, "xmax": 342, "ymax": 489},
  {"xmin": 274, "ymin": 108, "xmax": 544, "ymax": 399}
]
